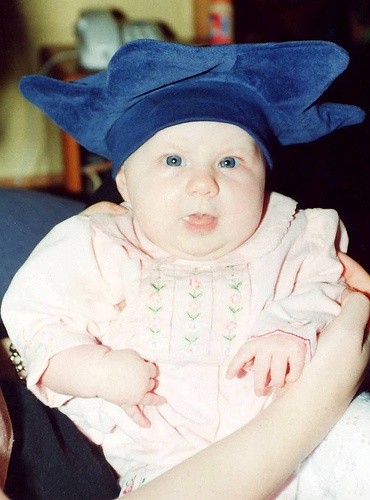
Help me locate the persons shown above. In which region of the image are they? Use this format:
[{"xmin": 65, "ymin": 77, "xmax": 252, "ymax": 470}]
[
  {"xmin": 0, "ymin": 34, "xmax": 369, "ymax": 500},
  {"xmin": 0, "ymin": 246, "xmax": 367, "ymax": 500}
]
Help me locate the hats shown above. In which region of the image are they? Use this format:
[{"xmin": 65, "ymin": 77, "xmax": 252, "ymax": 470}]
[{"xmin": 18, "ymin": 39, "xmax": 367, "ymax": 184}]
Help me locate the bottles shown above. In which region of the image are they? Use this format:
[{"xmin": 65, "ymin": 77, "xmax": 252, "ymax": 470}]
[{"xmin": 210, "ymin": 0, "xmax": 233, "ymax": 45}]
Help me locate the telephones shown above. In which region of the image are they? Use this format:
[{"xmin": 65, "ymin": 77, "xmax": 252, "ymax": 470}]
[{"xmin": 73, "ymin": 8, "xmax": 169, "ymax": 73}]
